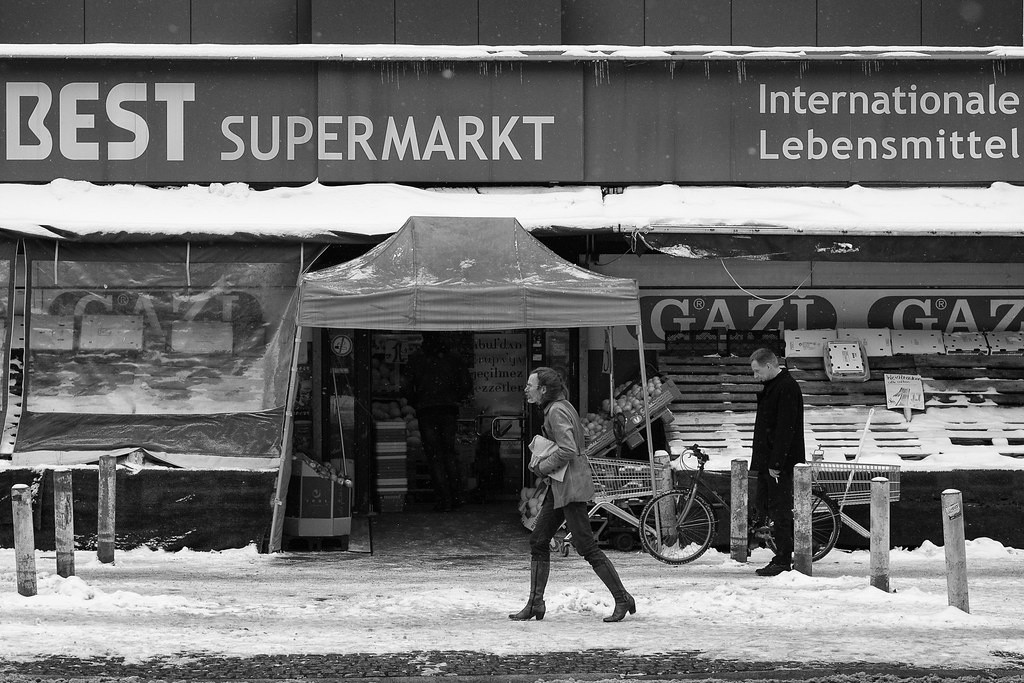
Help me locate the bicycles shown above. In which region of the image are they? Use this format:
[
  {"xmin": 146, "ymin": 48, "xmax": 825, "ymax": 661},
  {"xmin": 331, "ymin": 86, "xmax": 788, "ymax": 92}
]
[{"xmin": 639, "ymin": 444, "xmax": 841, "ymax": 562}]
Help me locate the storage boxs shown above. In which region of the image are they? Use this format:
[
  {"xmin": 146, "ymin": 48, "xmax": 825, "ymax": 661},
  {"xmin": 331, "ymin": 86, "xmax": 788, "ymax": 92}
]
[{"xmin": 580, "ymin": 362, "xmax": 682, "ymax": 458}]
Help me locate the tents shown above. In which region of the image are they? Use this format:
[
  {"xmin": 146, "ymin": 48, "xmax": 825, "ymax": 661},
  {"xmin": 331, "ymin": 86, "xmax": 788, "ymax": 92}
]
[{"xmin": 266, "ymin": 216, "xmax": 662, "ymax": 555}]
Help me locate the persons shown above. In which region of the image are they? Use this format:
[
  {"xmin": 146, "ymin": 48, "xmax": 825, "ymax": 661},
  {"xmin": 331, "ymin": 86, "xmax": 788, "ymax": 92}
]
[
  {"xmin": 509, "ymin": 366, "xmax": 636, "ymax": 623},
  {"xmin": 750, "ymin": 348, "xmax": 806, "ymax": 575}
]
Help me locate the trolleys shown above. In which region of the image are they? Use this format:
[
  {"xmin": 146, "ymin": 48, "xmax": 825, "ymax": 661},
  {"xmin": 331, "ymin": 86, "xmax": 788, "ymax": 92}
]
[
  {"xmin": 803, "ymin": 461, "xmax": 901, "ymax": 551},
  {"xmin": 543, "ymin": 450, "xmax": 663, "ymax": 558}
]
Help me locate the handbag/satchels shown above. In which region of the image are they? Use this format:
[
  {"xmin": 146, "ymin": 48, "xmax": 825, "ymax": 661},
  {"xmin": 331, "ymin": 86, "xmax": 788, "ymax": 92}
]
[{"xmin": 528, "ymin": 435, "xmax": 569, "ymax": 482}]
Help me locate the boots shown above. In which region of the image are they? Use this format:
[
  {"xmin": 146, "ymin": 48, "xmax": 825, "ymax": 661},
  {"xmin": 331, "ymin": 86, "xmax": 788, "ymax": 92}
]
[
  {"xmin": 509, "ymin": 561, "xmax": 550, "ymax": 621},
  {"xmin": 593, "ymin": 558, "xmax": 636, "ymax": 622}
]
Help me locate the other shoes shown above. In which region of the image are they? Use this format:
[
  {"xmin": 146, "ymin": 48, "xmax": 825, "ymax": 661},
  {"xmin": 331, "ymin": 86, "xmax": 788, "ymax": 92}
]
[{"xmin": 756, "ymin": 561, "xmax": 791, "ymax": 577}]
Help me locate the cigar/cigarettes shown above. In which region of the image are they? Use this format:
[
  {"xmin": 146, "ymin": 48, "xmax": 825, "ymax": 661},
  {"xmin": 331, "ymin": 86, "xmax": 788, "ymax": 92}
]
[{"xmin": 776, "ymin": 477, "xmax": 778, "ymax": 483}]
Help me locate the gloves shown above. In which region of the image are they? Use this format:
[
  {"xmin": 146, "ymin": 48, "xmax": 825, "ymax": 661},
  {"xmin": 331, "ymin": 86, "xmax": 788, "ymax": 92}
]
[{"xmin": 533, "ymin": 463, "xmax": 548, "ymax": 480}]
